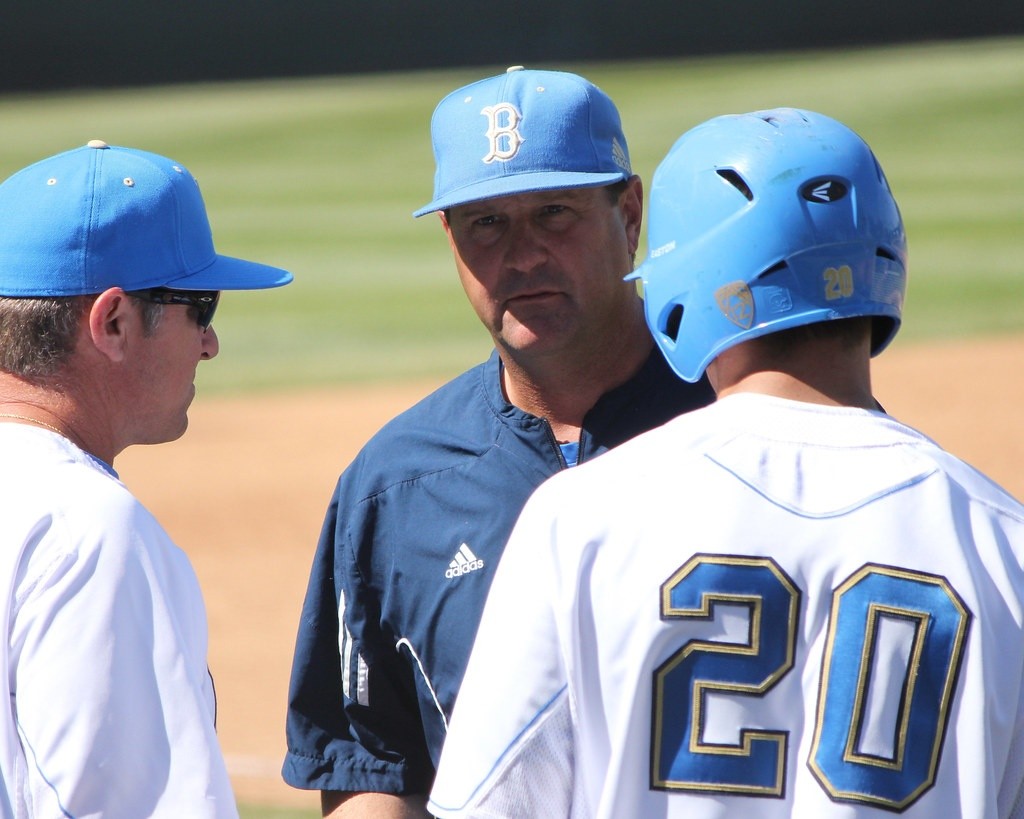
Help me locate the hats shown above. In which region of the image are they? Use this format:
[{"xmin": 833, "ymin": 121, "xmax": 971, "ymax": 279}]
[
  {"xmin": 0, "ymin": 139, "xmax": 292, "ymax": 295},
  {"xmin": 414, "ymin": 67, "xmax": 633, "ymax": 219}
]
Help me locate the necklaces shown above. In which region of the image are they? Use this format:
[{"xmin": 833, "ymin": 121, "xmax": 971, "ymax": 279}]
[{"xmin": 0, "ymin": 413, "xmax": 69, "ymax": 439}]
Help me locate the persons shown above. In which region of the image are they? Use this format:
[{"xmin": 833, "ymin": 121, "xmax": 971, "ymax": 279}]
[
  {"xmin": 278, "ymin": 66, "xmax": 890, "ymax": 819},
  {"xmin": 424, "ymin": 107, "xmax": 1024, "ymax": 819},
  {"xmin": 0, "ymin": 138, "xmax": 292, "ymax": 818}
]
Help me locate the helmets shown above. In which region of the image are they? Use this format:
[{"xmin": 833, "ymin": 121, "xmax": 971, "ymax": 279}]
[{"xmin": 623, "ymin": 108, "xmax": 905, "ymax": 383}]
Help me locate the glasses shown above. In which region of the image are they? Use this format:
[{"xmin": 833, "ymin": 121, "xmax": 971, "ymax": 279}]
[{"xmin": 128, "ymin": 290, "xmax": 221, "ymax": 326}]
